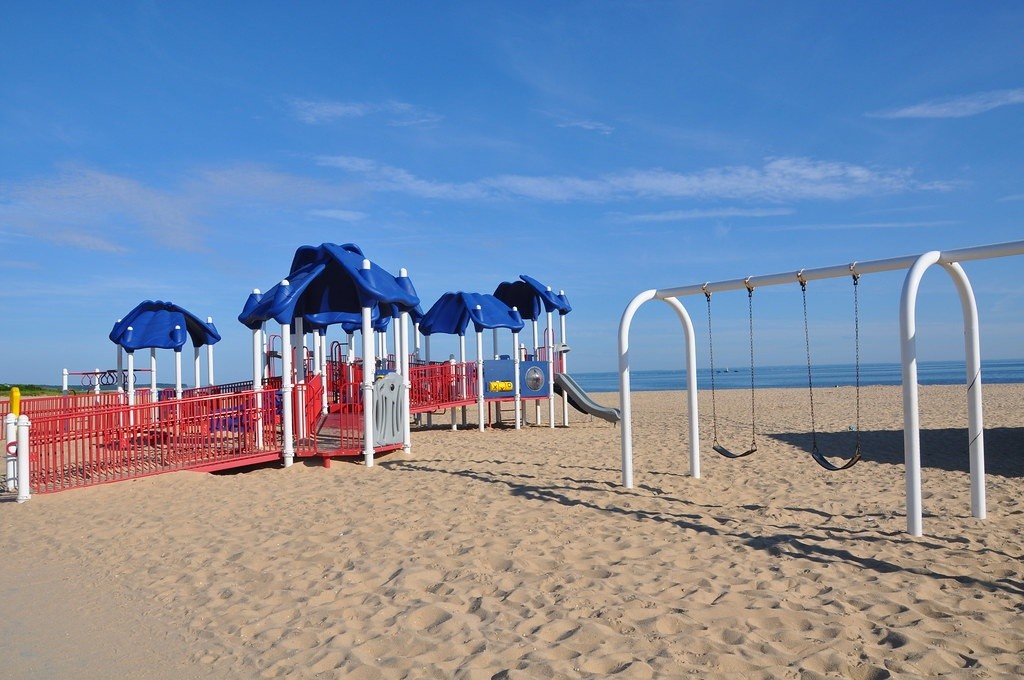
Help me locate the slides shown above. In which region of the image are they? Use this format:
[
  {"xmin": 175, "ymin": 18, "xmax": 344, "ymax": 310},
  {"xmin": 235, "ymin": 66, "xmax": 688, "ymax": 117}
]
[{"xmin": 556, "ymin": 373, "xmax": 621, "ymax": 422}]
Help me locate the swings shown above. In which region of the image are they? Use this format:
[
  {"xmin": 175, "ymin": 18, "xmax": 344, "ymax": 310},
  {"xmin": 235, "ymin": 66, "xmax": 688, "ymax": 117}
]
[
  {"xmin": 706, "ymin": 291, "xmax": 758, "ymax": 458},
  {"xmin": 800, "ymin": 278, "xmax": 862, "ymax": 471}
]
